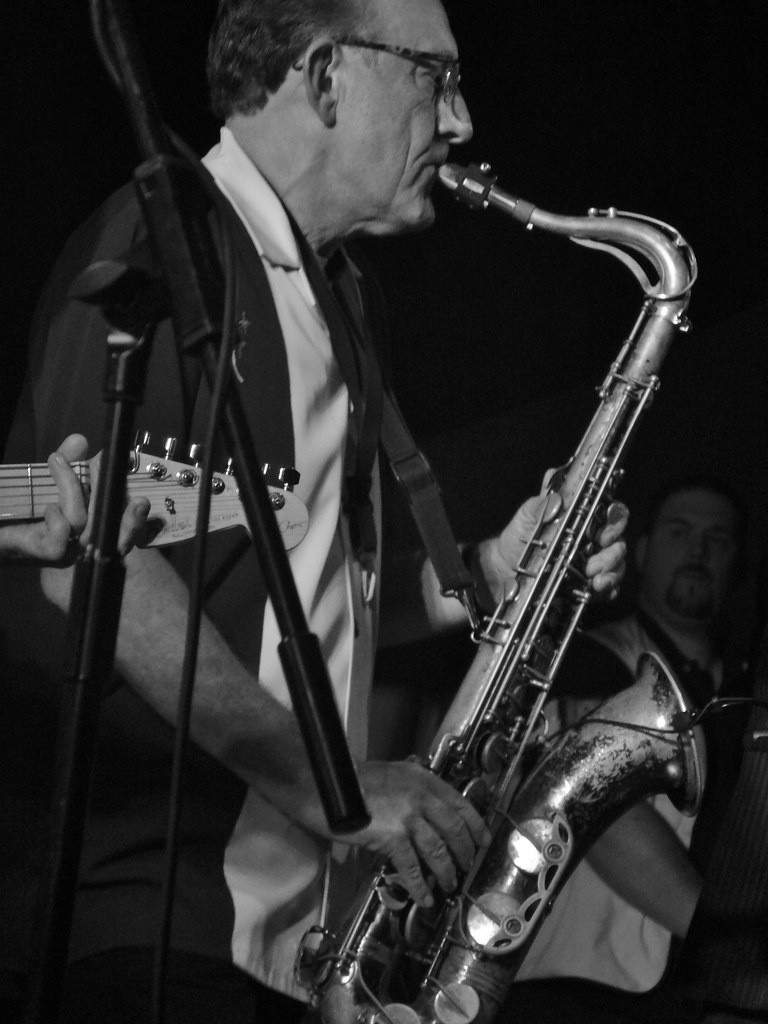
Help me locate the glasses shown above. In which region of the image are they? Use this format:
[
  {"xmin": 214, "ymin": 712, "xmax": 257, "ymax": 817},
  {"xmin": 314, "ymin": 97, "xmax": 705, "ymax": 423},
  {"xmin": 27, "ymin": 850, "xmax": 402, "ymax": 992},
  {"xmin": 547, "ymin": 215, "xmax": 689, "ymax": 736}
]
[{"xmin": 292, "ymin": 34, "xmax": 464, "ymax": 103}]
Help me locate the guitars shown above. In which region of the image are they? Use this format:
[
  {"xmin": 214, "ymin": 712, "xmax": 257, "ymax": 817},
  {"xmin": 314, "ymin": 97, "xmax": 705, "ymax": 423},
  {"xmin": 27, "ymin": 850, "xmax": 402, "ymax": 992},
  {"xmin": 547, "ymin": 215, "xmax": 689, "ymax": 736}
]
[{"xmin": 2, "ymin": 424, "xmax": 313, "ymax": 556}]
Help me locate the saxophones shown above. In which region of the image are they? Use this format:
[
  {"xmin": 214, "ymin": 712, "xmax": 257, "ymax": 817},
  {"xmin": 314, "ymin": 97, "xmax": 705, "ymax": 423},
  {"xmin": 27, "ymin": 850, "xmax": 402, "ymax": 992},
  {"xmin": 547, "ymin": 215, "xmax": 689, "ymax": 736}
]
[{"xmin": 295, "ymin": 162, "xmax": 710, "ymax": 1024}]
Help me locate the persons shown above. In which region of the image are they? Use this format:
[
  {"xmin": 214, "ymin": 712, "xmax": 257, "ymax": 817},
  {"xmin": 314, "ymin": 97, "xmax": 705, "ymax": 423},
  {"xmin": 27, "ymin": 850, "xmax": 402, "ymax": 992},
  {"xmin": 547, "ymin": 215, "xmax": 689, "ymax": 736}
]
[
  {"xmin": 13, "ymin": 0, "xmax": 631, "ymax": 1024},
  {"xmin": 510, "ymin": 466, "xmax": 752, "ymax": 1024},
  {"xmin": 0, "ymin": 426, "xmax": 154, "ymax": 567}
]
